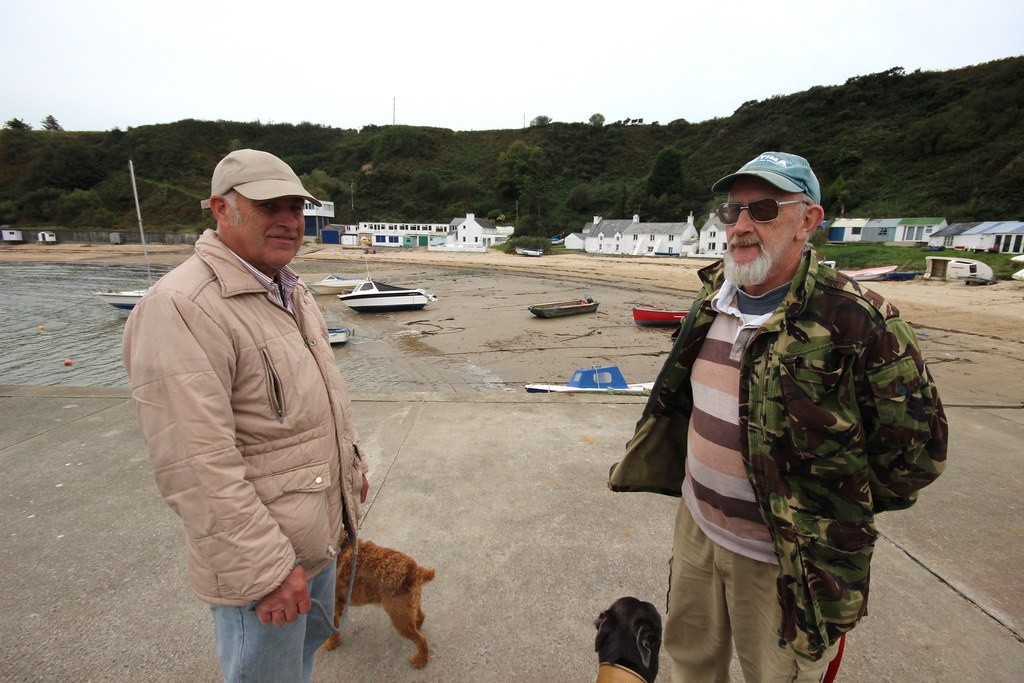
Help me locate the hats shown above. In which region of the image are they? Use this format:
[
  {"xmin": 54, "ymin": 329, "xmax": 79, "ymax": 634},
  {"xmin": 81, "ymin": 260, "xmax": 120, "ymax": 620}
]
[
  {"xmin": 711, "ymin": 150, "xmax": 820, "ymax": 210},
  {"xmin": 201, "ymin": 147, "xmax": 322, "ymax": 209}
]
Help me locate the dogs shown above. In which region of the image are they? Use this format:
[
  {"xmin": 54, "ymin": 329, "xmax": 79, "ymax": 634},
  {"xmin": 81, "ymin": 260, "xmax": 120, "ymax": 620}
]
[
  {"xmin": 594, "ymin": 595, "xmax": 662, "ymax": 683},
  {"xmin": 323, "ymin": 532, "xmax": 435, "ymax": 671}
]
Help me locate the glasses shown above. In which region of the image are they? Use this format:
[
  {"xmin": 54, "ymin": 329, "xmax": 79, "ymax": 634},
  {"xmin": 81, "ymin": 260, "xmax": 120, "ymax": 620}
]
[{"xmin": 715, "ymin": 200, "xmax": 809, "ymax": 225}]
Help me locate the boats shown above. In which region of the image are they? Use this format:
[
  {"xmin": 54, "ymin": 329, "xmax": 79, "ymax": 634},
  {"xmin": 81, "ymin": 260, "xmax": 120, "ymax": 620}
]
[
  {"xmin": 93, "ymin": 158, "xmax": 155, "ymax": 310},
  {"xmin": 327, "ymin": 328, "xmax": 350, "ymax": 345},
  {"xmin": 523, "ymin": 364, "xmax": 656, "ymax": 397},
  {"xmin": 840, "ymin": 265, "xmax": 899, "ymax": 281},
  {"xmin": 923, "ymin": 257, "xmax": 994, "ymax": 284},
  {"xmin": 335, "ymin": 257, "xmax": 439, "ymax": 313},
  {"xmin": 310, "ymin": 273, "xmax": 365, "ymax": 295},
  {"xmin": 514, "ymin": 246, "xmax": 544, "ymax": 257},
  {"xmin": 654, "ymin": 252, "xmax": 680, "ymax": 257},
  {"xmin": 632, "ymin": 306, "xmax": 691, "ymax": 327},
  {"xmin": 527, "ymin": 297, "xmax": 601, "ymax": 319},
  {"xmin": 881, "ymin": 269, "xmax": 920, "ymax": 281}
]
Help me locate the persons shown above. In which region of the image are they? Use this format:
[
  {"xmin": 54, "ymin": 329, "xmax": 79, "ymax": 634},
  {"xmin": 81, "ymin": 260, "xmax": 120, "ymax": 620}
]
[
  {"xmin": 607, "ymin": 150, "xmax": 951, "ymax": 683},
  {"xmin": 123, "ymin": 146, "xmax": 372, "ymax": 683}
]
